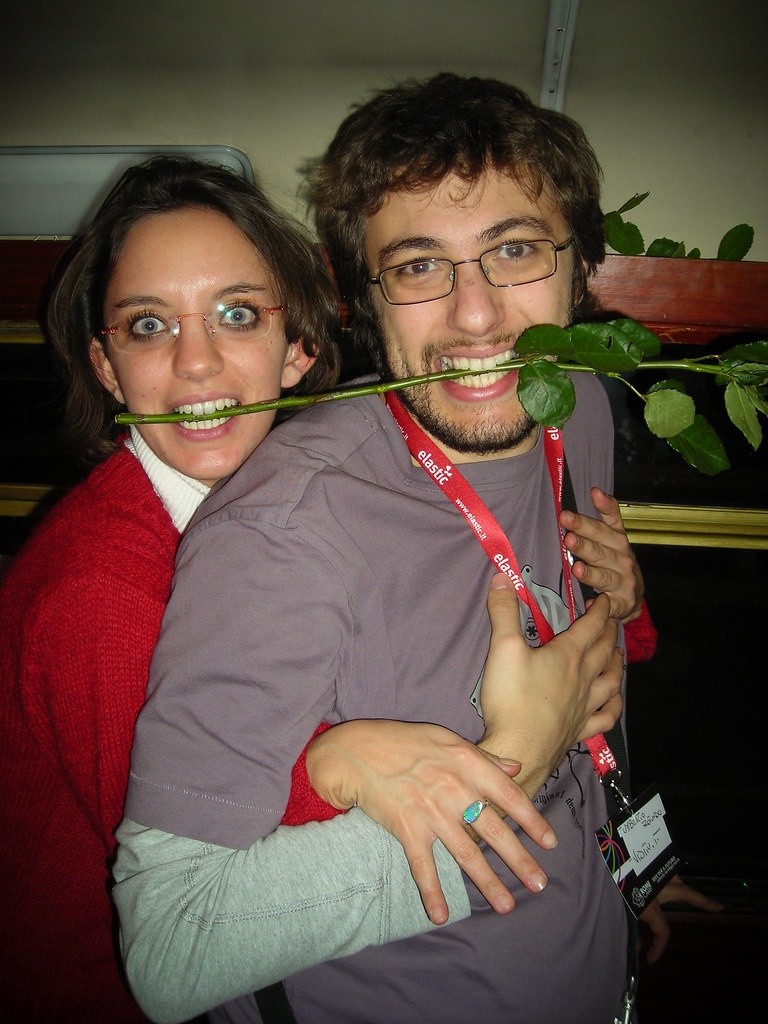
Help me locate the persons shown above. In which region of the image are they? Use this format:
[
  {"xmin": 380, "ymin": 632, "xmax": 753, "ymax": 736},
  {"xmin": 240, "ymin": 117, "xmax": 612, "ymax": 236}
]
[
  {"xmin": 113, "ymin": 71, "xmax": 724, "ymax": 1024},
  {"xmin": 0, "ymin": 146, "xmax": 656, "ymax": 1024}
]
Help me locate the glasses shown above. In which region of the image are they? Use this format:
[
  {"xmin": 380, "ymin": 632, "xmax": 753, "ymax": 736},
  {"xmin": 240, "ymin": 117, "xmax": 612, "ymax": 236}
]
[
  {"xmin": 100, "ymin": 303, "xmax": 285, "ymax": 353},
  {"xmin": 370, "ymin": 234, "xmax": 576, "ymax": 306}
]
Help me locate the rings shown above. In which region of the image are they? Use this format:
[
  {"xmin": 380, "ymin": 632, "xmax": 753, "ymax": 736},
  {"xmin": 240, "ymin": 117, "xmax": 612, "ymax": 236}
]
[{"xmin": 463, "ymin": 799, "xmax": 489, "ymax": 825}]
